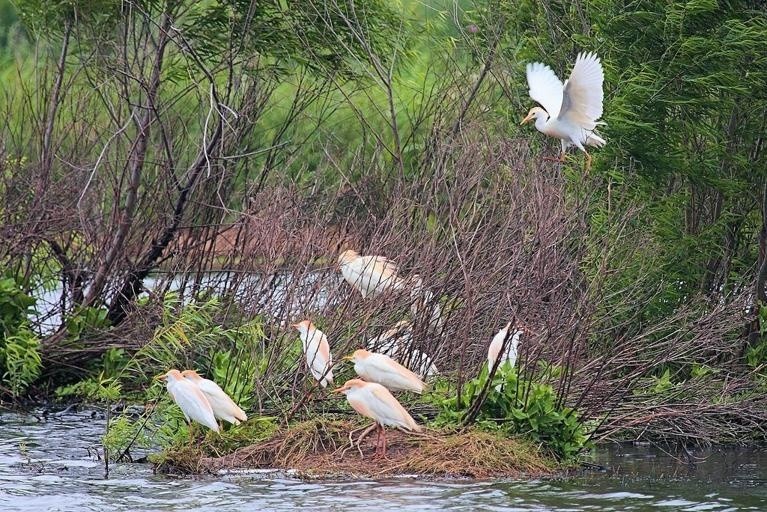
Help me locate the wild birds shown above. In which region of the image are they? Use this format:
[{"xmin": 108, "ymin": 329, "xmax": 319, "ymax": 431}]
[
  {"xmin": 335, "ymin": 249, "xmax": 407, "ymax": 301},
  {"xmin": 158, "ymin": 369, "xmax": 220, "ymax": 451},
  {"xmin": 292, "ymin": 319, "xmax": 334, "ymax": 397},
  {"xmin": 487, "ymin": 321, "xmax": 524, "ymax": 394},
  {"xmin": 332, "ymin": 320, "xmax": 440, "ymax": 437},
  {"xmin": 410, "ymin": 274, "xmax": 446, "ymax": 339},
  {"xmin": 519, "ymin": 52, "xmax": 608, "ymax": 178},
  {"xmin": 180, "ymin": 370, "xmax": 248, "ymax": 426}
]
